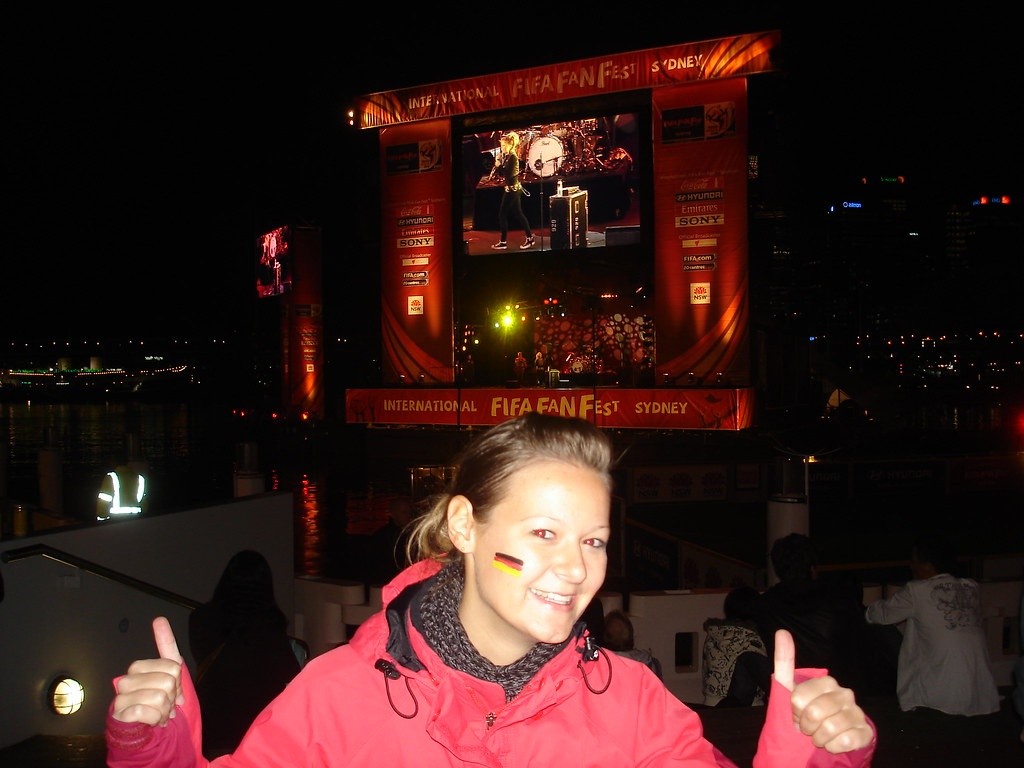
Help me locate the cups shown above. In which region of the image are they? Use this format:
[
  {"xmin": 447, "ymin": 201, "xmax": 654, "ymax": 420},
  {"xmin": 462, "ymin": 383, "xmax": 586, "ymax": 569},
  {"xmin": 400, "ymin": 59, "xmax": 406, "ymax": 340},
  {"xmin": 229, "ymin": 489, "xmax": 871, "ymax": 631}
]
[{"xmin": 563, "ymin": 189, "xmax": 568, "ymax": 196}]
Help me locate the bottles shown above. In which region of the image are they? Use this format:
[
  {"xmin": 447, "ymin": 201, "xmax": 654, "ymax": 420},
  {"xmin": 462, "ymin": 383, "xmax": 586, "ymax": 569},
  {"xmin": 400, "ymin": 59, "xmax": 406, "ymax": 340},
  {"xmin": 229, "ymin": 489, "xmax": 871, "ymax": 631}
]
[
  {"xmin": 556, "ymin": 178, "xmax": 562, "ymax": 196},
  {"xmin": 547, "ymin": 366, "xmax": 550, "ymax": 371}
]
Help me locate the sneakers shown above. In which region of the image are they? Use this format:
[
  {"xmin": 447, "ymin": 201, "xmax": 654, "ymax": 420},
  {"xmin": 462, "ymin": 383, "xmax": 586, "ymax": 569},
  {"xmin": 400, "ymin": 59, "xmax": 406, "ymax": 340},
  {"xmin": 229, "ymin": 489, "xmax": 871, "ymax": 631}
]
[
  {"xmin": 491, "ymin": 240, "xmax": 507, "ymax": 250},
  {"xmin": 519, "ymin": 237, "xmax": 535, "ymax": 249}
]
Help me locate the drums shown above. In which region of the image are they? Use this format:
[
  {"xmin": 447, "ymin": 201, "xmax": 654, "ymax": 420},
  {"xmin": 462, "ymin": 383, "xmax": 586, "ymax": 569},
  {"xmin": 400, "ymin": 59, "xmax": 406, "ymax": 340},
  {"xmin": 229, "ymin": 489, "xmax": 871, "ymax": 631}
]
[{"xmin": 528, "ymin": 135, "xmax": 564, "ymax": 177}]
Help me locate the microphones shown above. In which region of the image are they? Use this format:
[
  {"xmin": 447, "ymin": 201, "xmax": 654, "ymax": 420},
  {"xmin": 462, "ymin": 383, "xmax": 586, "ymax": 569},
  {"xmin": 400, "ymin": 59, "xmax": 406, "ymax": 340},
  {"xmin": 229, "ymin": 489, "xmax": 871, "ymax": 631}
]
[
  {"xmin": 497, "ymin": 148, "xmax": 503, "ymax": 160},
  {"xmin": 522, "ymin": 162, "xmax": 529, "ymax": 180}
]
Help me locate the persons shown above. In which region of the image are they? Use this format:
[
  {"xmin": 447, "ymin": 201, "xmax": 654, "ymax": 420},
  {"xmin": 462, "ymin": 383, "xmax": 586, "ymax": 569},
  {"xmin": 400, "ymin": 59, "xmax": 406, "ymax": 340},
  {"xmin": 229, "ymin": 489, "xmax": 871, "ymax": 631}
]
[
  {"xmin": 598, "ymin": 608, "xmax": 664, "ymax": 687},
  {"xmin": 490, "ymin": 129, "xmax": 536, "ymax": 252},
  {"xmin": 749, "ymin": 531, "xmax": 872, "ymax": 713},
  {"xmin": 514, "ymin": 350, "xmax": 528, "ymax": 384},
  {"xmin": 185, "ymin": 549, "xmax": 306, "ymax": 764},
  {"xmin": 97, "ymin": 409, "xmax": 883, "ymax": 768},
  {"xmin": 698, "ymin": 583, "xmax": 770, "ymax": 709},
  {"xmin": 533, "ymin": 350, "xmax": 550, "ymax": 388},
  {"xmin": 851, "ymin": 533, "xmax": 1001, "ymax": 734}
]
